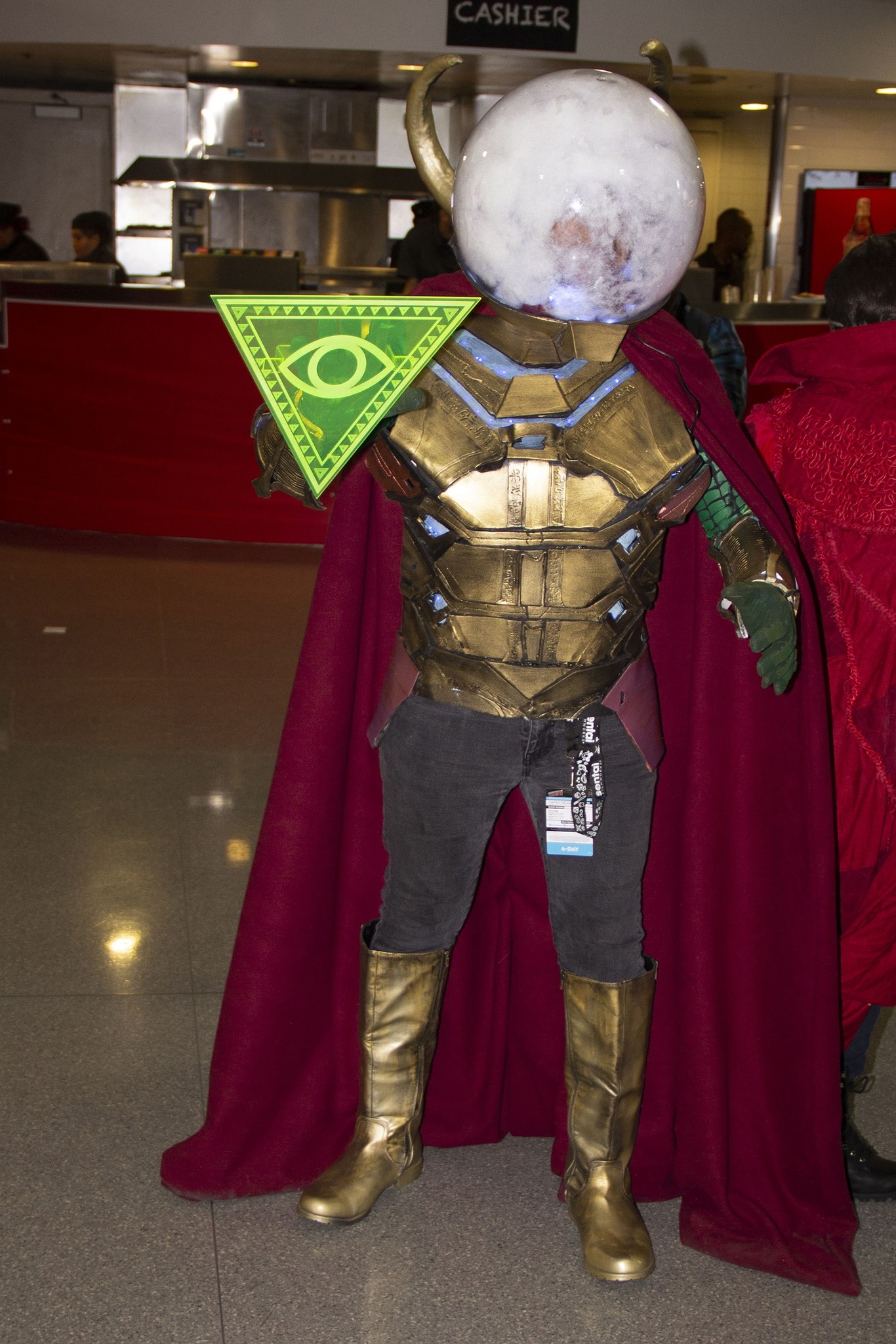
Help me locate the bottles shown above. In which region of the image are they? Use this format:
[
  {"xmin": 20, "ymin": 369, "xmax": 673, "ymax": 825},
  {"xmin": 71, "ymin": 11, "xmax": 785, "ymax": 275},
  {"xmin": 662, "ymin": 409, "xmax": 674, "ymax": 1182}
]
[{"xmin": 842, "ymin": 198, "xmax": 874, "ymax": 259}]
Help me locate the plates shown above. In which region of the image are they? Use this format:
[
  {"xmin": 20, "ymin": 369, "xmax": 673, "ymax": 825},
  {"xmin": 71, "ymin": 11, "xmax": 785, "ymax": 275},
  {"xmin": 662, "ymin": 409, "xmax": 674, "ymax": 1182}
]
[{"xmin": 791, "ymin": 294, "xmax": 825, "ymax": 301}]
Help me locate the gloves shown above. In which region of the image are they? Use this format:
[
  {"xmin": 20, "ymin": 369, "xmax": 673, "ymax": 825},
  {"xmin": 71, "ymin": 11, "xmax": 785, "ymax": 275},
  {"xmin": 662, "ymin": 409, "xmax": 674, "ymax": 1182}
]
[
  {"xmin": 380, "ymin": 386, "xmax": 425, "ymax": 420},
  {"xmin": 722, "ymin": 580, "xmax": 797, "ymax": 695}
]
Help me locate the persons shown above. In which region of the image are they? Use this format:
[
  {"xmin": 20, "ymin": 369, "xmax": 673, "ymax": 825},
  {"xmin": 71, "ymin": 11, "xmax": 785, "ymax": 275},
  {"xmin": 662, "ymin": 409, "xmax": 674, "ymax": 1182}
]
[
  {"xmin": 0, "ymin": 202, "xmax": 51, "ymax": 262},
  {"xmin": 678, "ymin": 208, "xmax": 752, "ymax": 311},
  {"xmin": 842, "ymin": 198, "xmax": 873, "ymax": 256},
  {"xmin": 71, "ymin": 211, "xmax": 128, "ymax": 283},
  {"xmin": 256, "ymin": 39, "xmax": 869, "ymax": 1297},
  {"xmin": 824, "ymin": 233, "xmax": 896, "ymax": 330},
  {"xmin": 387, "ymin": 199, "xmax": 456, "ymax": 295}
]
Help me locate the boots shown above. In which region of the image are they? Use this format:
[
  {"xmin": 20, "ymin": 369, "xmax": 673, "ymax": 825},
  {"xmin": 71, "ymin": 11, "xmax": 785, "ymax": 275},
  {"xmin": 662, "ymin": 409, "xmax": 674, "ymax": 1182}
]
[
  {"xmin": 296, "ymin": 924, "xmax": 448, "ymax": 1228},
  {"xmin": 558, "ymin": 954, "xmax": 654, "ymax": 1282}
]
